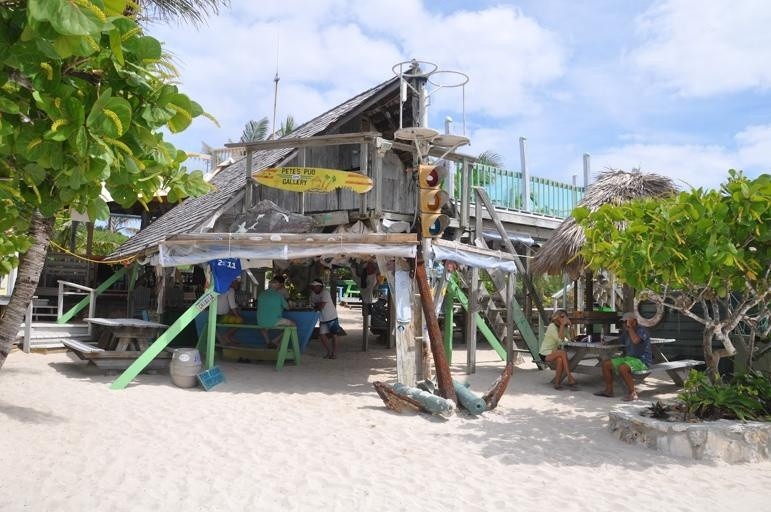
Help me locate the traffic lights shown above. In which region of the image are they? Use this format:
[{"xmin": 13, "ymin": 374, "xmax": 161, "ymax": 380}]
[{"xmin": 417, "ymin": 164, "xmax": 450, "ymax": 239}]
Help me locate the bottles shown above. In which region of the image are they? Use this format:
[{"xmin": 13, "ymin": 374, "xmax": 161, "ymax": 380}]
[{"xmin": 248, "ymin": 298, "xmax": 255, "ymax": 307}]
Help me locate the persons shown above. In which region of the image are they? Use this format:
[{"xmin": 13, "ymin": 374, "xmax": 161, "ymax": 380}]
[
  {"xmin": 593, "ymin": 312, "xmax": 653, "ymax": 402},
  {"xmin": 370, "ymin": 294, "xmax": 388, "ymax": 327},
  {"xmin": 218, "ymin": 279, "xmax": 245, "ymax": 345},
  {"xmin": 274, "ymin": 275, "xmax": 296, "ymax": 310},
  {"xmin": 538, "ymin": 309, "xmax": 579, "ymax": 391},
  {"xmin": 256, "ymin": 279, "xmax": 297, "ymax": 349},
  {"xmin": 308, "ymin": 278, "xmax": 340, "ymax": 359}
]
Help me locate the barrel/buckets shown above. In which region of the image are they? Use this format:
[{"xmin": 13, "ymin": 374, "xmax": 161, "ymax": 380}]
[{"xmin": 170, "ymin": 348, "xmax": 203, "ymax": 388}]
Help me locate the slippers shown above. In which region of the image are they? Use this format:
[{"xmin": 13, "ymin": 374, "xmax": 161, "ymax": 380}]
[
  {"xmin": 593, "ymin": 391, "xmax": 615, "ymax": 398},
  {"xmin": 554, "ymin": 382, "xmax": 581, "ymax": 392}
]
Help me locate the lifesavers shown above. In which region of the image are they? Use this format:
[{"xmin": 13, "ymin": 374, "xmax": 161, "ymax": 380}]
[{"xmin": 634, "ymin": 291, "xmax": 664, "ymax": 328}]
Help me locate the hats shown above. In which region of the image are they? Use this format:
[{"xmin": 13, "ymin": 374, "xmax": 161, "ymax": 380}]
[
  {"xmin": 308, "ymin": 281, "xmax": 322, "ymax": 286},
  {"xmin": 620, "ymin": 312, "xmax": 638, "ymax": 320}
]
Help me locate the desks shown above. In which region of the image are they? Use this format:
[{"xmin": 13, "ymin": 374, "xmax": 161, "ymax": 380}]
[
  {"xmin": 552, "ymin": 338, "xmax": 678, "ymax": 384},
  {"xmin": 83, "ymin": 318, "xmax": 169, "ymax": 351}
]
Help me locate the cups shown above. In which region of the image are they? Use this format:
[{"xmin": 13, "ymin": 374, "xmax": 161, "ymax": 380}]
[{"xmin": 289, "ymin": 298, "xmax": 313, "ymax": 308}]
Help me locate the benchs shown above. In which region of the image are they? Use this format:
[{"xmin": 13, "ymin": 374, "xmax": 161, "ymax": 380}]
[
  {"xmin": 531, "ymin": 352, "xmax": 624, "ymax": 369},
  {"xmin": 196, "ymin": 324, "xmax": 303, "ymax": 371},
  {"xmin": 59, "ymin": 337, "xmax": 104, "ymax": 362},
  {"xmin": 631, "ymin": 359, "xmax": 705, "ymax": 389}
]
